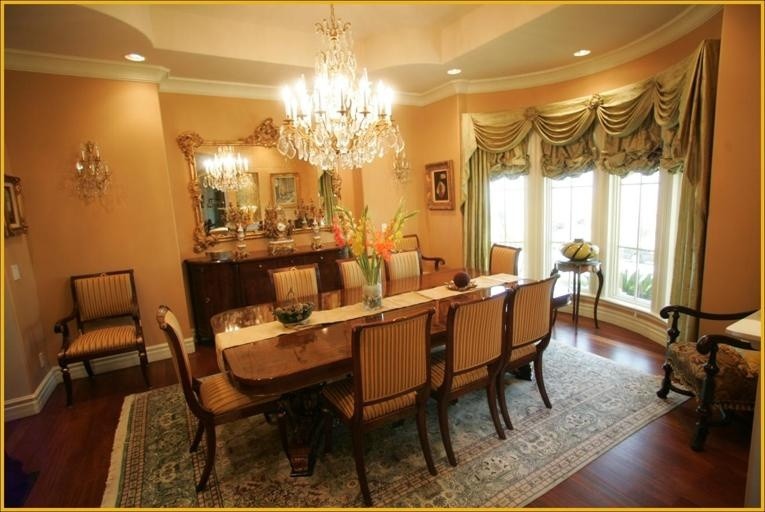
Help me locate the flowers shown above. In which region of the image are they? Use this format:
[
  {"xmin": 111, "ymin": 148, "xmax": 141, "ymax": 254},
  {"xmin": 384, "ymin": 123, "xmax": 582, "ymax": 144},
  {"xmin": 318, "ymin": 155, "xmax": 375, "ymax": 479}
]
[{"xmin": 328, "ymin": 195, "xmax": 420, "ymax": 286}]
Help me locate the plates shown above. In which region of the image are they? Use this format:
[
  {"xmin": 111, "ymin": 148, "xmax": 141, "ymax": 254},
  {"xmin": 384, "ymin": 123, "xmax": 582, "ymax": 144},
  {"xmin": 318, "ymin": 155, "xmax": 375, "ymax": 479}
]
[{"xmin": 444, "ymin": 279, "xmax": 477, "ymax": 291}]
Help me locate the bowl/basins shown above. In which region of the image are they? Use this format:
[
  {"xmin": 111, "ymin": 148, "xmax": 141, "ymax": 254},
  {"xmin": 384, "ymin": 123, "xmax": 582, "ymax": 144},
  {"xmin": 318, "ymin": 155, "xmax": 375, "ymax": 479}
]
[
  {"xmin": 205, "ymin": 250, "xmax": 232, "ymax": 262},
  {"xmin": 274, "ymin": 301, "xmax": 314, "ymax": 324}
]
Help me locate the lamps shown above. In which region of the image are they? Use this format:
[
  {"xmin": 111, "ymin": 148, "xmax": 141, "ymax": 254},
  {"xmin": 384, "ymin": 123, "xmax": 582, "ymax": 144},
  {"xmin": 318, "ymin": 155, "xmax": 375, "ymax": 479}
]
[
  {"xmin": 68, "ymin": 139, "xmax": 113, "ymax": 198},
  {"xmin": 269, "ymin": 3, "xmax": 407, "ymax": 178},
  {"xmin": 392, "ymin": 155, "xmax": 409, "ymax": 181},
  {"xmin": 200, "ymin": 144, "xmax": 254, "ymax": 194}
]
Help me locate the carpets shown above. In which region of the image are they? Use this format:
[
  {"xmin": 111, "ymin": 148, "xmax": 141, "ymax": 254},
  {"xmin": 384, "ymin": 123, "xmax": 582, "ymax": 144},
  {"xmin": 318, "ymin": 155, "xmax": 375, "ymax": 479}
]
[{"xmin": 97, "ymin": 331, "xmax": 698, "ymax": 510}]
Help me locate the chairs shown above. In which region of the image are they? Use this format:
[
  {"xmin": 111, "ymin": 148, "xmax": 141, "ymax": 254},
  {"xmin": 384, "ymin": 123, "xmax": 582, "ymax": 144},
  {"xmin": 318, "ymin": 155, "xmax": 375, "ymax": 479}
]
[
  {"xmin": 53, "ymin": 267, "xmax": 153, "ymax": 409},
  {"xmin": 657, "ymin": 305, "xmax": 762, "ymax": 453}
]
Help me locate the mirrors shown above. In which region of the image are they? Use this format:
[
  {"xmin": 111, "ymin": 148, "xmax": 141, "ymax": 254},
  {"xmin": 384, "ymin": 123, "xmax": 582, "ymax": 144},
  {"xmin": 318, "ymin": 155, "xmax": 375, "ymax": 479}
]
[{"xmin": 175, "ymin": 117, "xmax": 345, "ymax": 255}]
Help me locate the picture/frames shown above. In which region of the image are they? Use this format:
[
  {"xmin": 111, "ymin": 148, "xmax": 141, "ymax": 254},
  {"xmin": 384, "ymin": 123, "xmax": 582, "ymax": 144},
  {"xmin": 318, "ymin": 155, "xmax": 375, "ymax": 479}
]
[
  {"xmin": 268, "ymin": 170, "xmax": 304, "ymax": 212},
  {"xmin": 3, "ymin": 173, "xmax": 28, "ymax": 240},
  {"xmin": 422, "ymin": 158, "xmax": 457, "ymax": 211}
]
